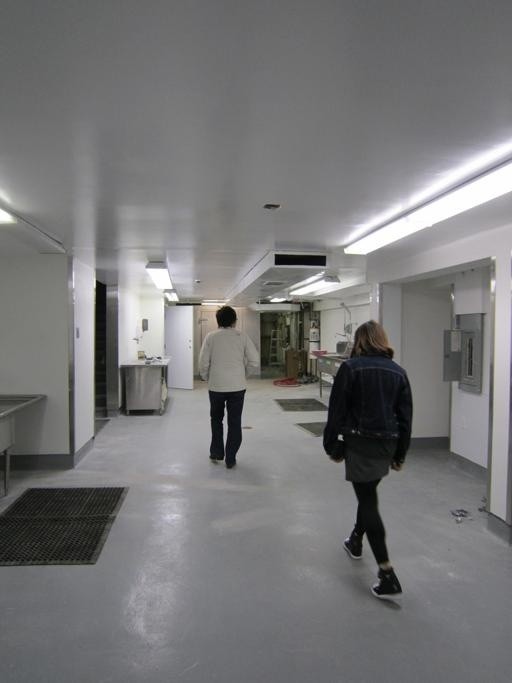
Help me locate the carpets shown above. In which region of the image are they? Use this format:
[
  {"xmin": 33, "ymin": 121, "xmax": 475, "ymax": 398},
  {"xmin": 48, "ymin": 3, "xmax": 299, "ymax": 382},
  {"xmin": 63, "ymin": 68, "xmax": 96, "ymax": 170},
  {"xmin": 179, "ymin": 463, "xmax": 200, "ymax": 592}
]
[
  {"xmin": 0, "ymin": 485, "xmax": 138, "ymax": 567},
  {"xmin": 273, "ymin": 398, "xmax": 331, "ymax": 436}
]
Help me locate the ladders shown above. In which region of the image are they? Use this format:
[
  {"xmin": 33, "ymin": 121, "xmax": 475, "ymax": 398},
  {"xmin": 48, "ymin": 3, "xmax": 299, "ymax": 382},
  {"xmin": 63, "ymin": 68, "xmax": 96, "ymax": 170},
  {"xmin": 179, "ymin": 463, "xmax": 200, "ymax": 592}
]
[{"xmin": 269, "ymin": 330, "xmax": 283, "ymax": 367}]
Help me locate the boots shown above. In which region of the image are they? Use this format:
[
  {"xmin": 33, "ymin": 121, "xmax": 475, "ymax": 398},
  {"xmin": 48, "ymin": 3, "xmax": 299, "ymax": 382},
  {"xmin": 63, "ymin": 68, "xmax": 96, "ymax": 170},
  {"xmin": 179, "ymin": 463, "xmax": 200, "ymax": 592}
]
[
  {"xmin": 343, "ymin": 529, "xmax": 364, "ymax": 560},
  {"xmin": 370, "ymin": 567, "xmax": 404, "ymax": 601}
]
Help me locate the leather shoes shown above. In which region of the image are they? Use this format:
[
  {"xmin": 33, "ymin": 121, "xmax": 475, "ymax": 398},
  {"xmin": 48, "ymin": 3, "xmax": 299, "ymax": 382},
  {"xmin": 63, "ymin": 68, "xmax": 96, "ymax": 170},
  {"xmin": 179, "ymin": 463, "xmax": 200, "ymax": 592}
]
[
  {"xmin": 209, "ymin": 454, "xmax": 224, "ymax": 460},
  {"xmin": 226, "ymin": 460, "xmax": 236, "ymax": 468}
]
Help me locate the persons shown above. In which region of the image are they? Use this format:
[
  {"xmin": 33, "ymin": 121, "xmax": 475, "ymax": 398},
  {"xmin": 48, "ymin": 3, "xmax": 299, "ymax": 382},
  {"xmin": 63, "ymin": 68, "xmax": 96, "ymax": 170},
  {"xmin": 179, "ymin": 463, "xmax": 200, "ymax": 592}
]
[
  {"xmin": 323, "ymin": 319, "xmax": 413, "ymax": 600},
  {"xmin": 197, "ymin": 302, "xmax": 261, "ymax": 470}
]
